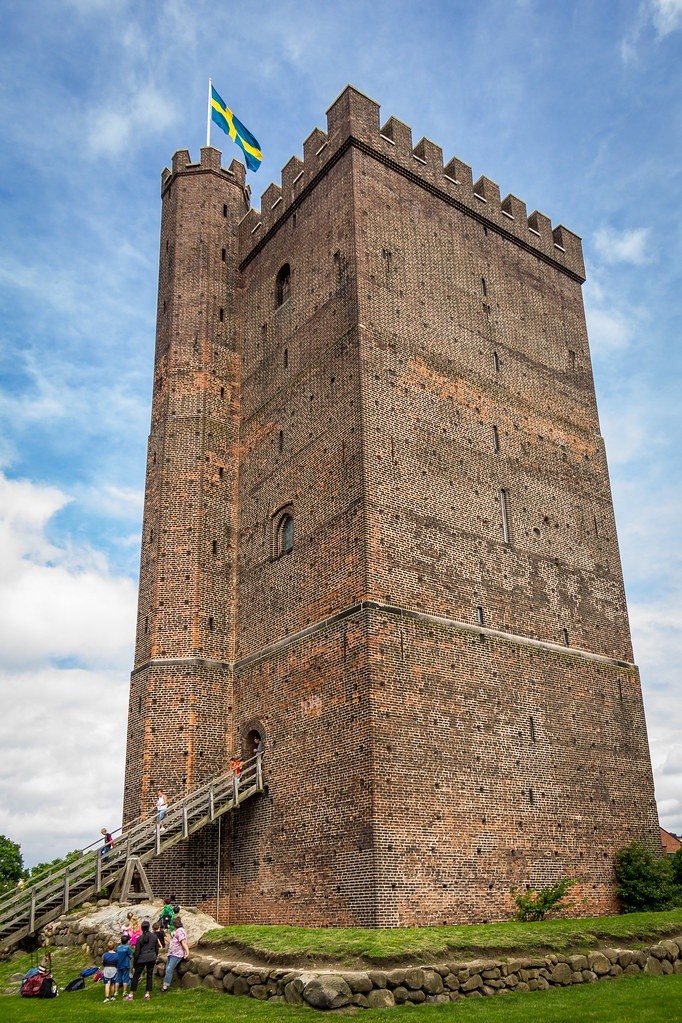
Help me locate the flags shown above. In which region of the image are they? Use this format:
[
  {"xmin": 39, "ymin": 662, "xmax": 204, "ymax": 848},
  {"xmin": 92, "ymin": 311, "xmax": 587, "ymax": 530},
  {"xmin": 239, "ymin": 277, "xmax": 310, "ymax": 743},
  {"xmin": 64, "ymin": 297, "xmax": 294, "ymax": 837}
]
[{"xmin": 209, "ymin": 85, "xmax": 262, "ymax": 175}]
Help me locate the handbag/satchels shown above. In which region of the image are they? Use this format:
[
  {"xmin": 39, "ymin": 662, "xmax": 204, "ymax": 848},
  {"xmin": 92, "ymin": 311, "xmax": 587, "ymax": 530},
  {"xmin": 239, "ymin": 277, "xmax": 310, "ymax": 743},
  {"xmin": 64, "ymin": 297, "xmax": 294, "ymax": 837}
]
[{"xmin": 158, "ymin": 914, "xmax": 172, "ymax": 929}]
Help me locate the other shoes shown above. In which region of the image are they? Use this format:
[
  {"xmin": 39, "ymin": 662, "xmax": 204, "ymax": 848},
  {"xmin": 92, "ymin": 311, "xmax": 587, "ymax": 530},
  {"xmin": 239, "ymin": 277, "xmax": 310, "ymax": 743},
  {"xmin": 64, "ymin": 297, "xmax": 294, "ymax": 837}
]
[
  {"xmin": 123, "ymin": 992, "xmax": 128, "ymax": 996},
  {"xmin": 102, "ymin": 861, "xmax": 109, "ymax": 865},
  {"xmin": 160, "ymin": 986, "xmax": 168, "ymax": 992},
  {"xmin": 114, "ymin": 992, "xmax": 118, "ymax": 997},
  {"xmin": 123, "ymin": 994, "xmax": 133, "ymax": 1000},
  {"xmin": 154, "ymin": 828, "xmax": 165, "ymax": 833},
  {"xmin": 111, "ymin": 997, "xmax": 116, "ymax": 1001},
  {"xmin": 144, "ymin": 994, "xmax": 150, "ymax": 1000},
  {"xmin": 103, "ymin": 998, "xmax": 110, "ymax": 1003}
]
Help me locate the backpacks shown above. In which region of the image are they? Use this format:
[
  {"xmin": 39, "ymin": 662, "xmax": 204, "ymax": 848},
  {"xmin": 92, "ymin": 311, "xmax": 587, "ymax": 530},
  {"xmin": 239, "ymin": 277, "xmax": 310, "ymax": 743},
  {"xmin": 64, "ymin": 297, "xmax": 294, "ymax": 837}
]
[{"xmin": 65, "ymin": 977, "xmax": 85, "ymax": 990}]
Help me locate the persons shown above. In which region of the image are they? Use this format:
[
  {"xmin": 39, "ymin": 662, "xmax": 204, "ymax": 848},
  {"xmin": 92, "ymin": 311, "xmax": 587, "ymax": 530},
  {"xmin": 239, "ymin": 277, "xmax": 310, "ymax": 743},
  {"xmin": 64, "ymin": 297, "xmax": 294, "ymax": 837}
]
[
  {"xmin": 101, "ymin": 940, "xmax": 120, "ymax": 1003},
  {"xmin": 252, "ymin": 734, "xmax": 264, "ymax": 760},
  {"xmin": 122, "ymin": 921, "xmax": 160, "ymax": 1000},
  {"xmin": 160, "ymin": 897, "xmax": 177, "ymax": 932},
  {"xmin": 158, "ymin": 917, "xmax": 189, "ymax": 993},
  {"xmin": 228, "ymin": 757, "xmax": 243, "ymax": 786},
  {"xmin": 122, "ymin": 914, "xmax": 143, "ymax": 949},
  {"xmin": 113, "ymin": 935, "xmax": 134, "ymax": 998},
  {"xmin": 154, "ymin": 788, "xmax": 167, "ymax": 835},
  {"xmin": 100, "ymin": 827, "xmax": 114, "ymax": 865},
  {"xmin": 117, "ymin": 912, "xmax": 134, "ymax": 936},
  {"xmin": 150, "ymin": 923, "xmax": 172, "ymax": 965},
  {"xmin": 169, "ymin": 906, "xmax": 183, "ymax": 930}
]
